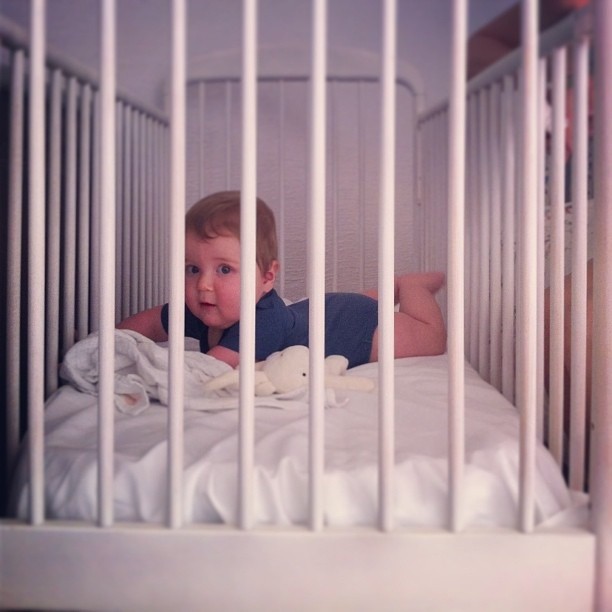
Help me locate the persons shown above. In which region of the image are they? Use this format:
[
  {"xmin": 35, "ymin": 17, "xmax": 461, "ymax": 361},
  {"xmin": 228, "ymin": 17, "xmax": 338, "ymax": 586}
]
[{"xmin": 115, "ymin": 190, "xmax": 446, "ymax": 370}]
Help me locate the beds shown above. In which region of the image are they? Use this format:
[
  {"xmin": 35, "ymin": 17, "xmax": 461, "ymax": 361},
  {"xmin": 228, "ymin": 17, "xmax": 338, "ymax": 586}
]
[{"xmin": 0, "ymin": 0, "xmax": 612, "ymax": 611}]
